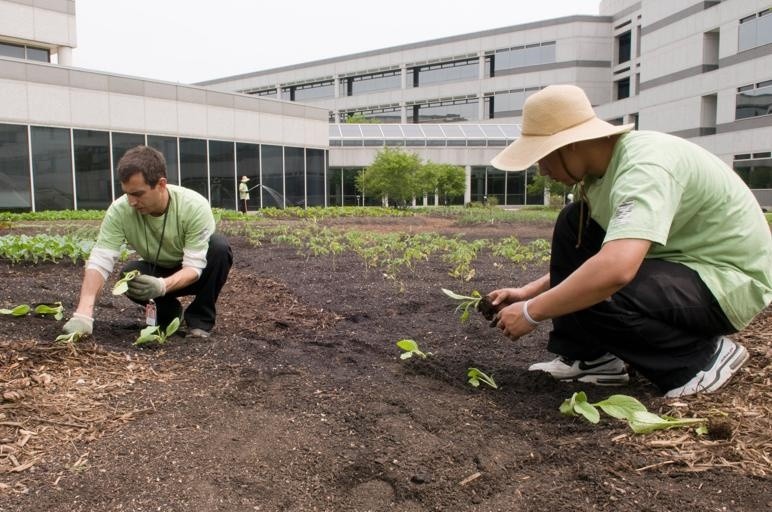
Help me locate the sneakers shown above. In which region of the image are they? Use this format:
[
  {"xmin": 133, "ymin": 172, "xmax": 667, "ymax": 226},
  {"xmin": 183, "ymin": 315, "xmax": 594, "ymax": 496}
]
[
  {"xmin": 666, "ymin": 335, "xmax": 750, "ymax": 399},
  {"xmin": 529, "ymin": 351, "xmax": 629, "ymax": 386},
  {"xmin": 186, "ymin": 326, "xmax": 210, "ymax": 339}
]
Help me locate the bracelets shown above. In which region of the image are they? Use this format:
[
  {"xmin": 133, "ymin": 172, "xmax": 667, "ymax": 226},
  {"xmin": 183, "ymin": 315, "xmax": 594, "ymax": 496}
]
[{"xmin": 522, "ymin": 301, "xmax": 539, "ymax": 326}]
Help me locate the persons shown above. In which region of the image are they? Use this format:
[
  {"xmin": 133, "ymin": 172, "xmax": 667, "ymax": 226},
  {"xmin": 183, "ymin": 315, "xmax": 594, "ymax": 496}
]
[
  {"xmin": 239, "ymin": 174, "xmax": 252, "ymax": 210},
  {"xmin": 62, "ymin": 147, "xmax": 234, "ymax": 342},
  {"xmin": 479, "ymin": 85, "xmax": 772, "ymax": 398}
]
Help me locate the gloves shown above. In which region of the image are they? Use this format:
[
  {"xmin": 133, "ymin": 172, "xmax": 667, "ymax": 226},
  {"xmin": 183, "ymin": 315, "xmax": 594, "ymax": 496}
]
[
  {"xmin": 125, "ymin": 275, "xmax": 166, "ymax": 300},
  {"xmin": 62, "ymin": 313, "xmax": 94, "ymax": 336}
]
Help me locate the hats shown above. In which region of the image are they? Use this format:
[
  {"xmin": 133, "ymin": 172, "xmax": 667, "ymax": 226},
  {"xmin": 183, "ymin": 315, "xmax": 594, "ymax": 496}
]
[
  {"xmin": 241, "ymin": 176, "xmax": 249, "ymax": 182},
  {"xmin": 491, "ymin": 85, "xmax": 635, "ymax": 171}
]
